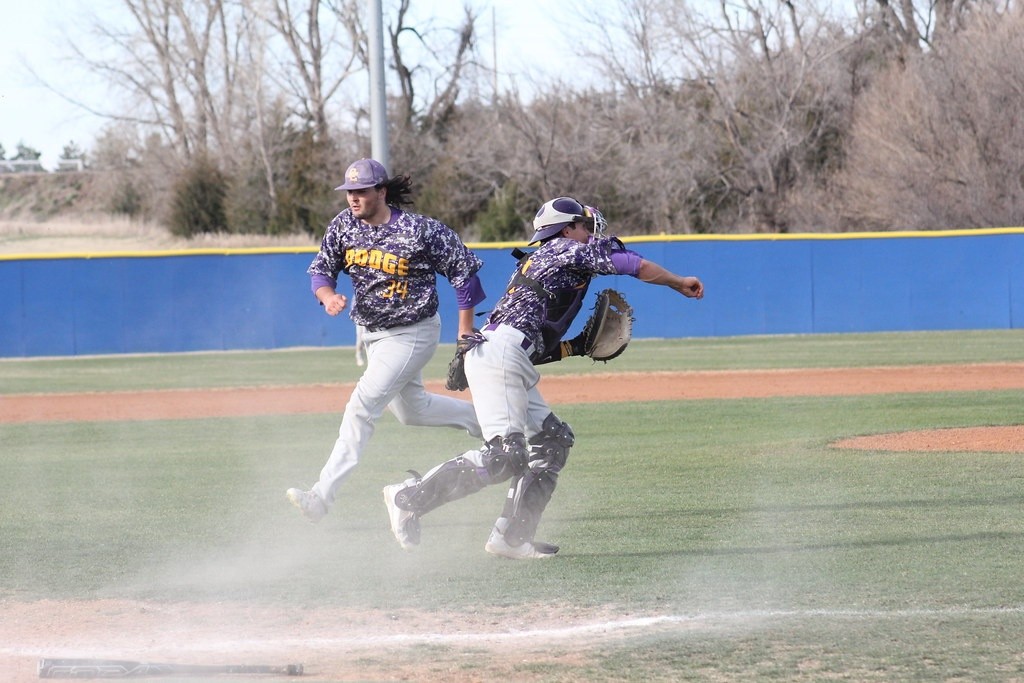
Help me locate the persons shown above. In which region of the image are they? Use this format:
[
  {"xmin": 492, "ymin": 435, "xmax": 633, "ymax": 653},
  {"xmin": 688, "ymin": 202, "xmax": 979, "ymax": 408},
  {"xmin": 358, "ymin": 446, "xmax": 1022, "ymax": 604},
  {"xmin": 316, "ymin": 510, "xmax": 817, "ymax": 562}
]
[
  {"xmin": 383, "ymin": 196, "xmax": 704, "ymax": 557},
  {"xmin": 287, "ymin": 159, "xmax": 490, "ymax": 527}
]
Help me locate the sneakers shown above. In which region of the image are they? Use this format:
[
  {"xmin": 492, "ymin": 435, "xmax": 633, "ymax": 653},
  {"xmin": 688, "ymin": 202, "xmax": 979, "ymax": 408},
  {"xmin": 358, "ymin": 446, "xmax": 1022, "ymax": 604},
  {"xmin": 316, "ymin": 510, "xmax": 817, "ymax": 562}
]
[
  {"xmin": 382, "ymin": 477, "xmax": 421, "ymax": 553},
  {"xmin": 286, "ymin": 487, "xmax": 328, "ymax": 525},
  {"xmin": 484, "ymin": 516, "xmax": 560, "ymax": 559}
]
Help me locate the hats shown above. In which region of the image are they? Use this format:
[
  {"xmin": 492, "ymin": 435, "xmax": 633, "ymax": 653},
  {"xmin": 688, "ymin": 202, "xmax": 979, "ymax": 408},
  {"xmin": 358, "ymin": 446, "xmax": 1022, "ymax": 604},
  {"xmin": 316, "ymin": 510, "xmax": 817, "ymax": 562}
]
[{"xmin": 333, "ymin": 157, "xmax": 389, "ymax": 191}]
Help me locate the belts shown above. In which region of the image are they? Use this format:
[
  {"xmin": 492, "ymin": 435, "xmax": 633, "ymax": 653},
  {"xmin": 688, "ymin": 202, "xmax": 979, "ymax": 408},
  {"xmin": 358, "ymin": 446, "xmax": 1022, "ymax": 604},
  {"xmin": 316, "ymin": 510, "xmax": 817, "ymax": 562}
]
[{"xmin": 483, "ymin": 323, "xmax": 537, "ymax": 363}]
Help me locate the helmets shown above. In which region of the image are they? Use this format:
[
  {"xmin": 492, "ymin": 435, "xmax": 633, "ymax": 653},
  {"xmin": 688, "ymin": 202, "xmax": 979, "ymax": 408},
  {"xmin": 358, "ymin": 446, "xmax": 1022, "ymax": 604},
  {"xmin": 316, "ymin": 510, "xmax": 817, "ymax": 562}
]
[{"xmin": 526, "ymin": 196, "xmax": 595, "ymax": 246}]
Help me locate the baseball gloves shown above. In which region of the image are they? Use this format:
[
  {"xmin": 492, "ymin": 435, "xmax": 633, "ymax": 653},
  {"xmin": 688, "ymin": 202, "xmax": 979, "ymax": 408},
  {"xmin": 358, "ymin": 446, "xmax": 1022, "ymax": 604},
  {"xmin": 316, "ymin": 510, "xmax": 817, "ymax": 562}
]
[
  {"xmin": 445, "ymin": 339, "xmax": 479, "ymax": 392},
  {"xmin": 583, "ymin": 287, "xmax": 633, "ymax": 361}
]
